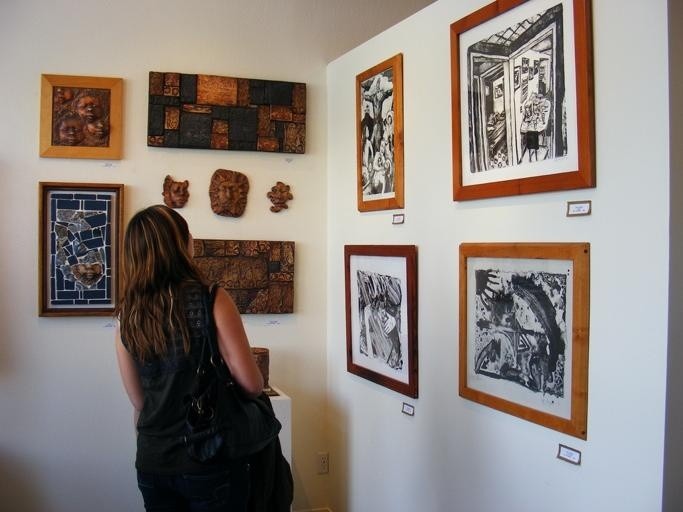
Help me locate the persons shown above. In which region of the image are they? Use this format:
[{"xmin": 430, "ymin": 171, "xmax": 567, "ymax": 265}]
[
  {"xmin": 359, "ymin": 74, "xmax": 397, "ymax": 202},
  {"xmin": 107, "ymin": 202, "xmax": 296, "ymax": 511},
  {"xmin": 266, "ymin": 181, "xmax": 292, "ymax": 212},
  {"xmin": 53, "ymin": 88, "xmax": 72, "ymax": 106},
  {"xmin": 55, "ymin": 116, "xmax": 81, "ymax": 145},
  {"xmin": 87, "ymin": 120, "xmax": 108, "ymax": 139},
  {"xmin": 161, "ymin": 173, "xmax": 189, "ymax": 208},
  {"xmin": 71, "ymin": 90, "xmax": 104, "ymax": 122},
  {"xmin": 72, "ymin": 261, "xmax": 103, "ymax": 279},
  {"xmin": 207, "ymin": 168, "xmax": 248, "ymax": 217}
]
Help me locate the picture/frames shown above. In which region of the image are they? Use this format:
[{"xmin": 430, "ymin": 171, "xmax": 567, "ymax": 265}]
[
  {"xmin": 450, "ymin": 0, "xmax": 596, "ymax": 201},
  {"xmin": 355, "ymin": 53, "xmax": 406, "ymax": 212},
  {"xmin": 39, "ymin": 74, "xmax": 123, "ymax": 160},
  {"xmin": 345, "ymin": 245, "xmax": 418, "ymax": 399},
  {"xmin": 38, "ymin": 181, "xmax": 125, "ymax": 317},
  {"xmin": 459, "ymin": 243, "xmax": 591, "ymax": 441}
]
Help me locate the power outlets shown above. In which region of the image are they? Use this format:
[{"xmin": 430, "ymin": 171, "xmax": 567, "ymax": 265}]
[{"xmin": 316, "ymin": 452, "xmax": 329, "ymax": 474}]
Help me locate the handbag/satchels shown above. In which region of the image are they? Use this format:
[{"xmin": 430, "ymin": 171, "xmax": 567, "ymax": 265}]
[{"xmin": 185, "ymin": 378, "xmax": 282, "ymax": 478}]
